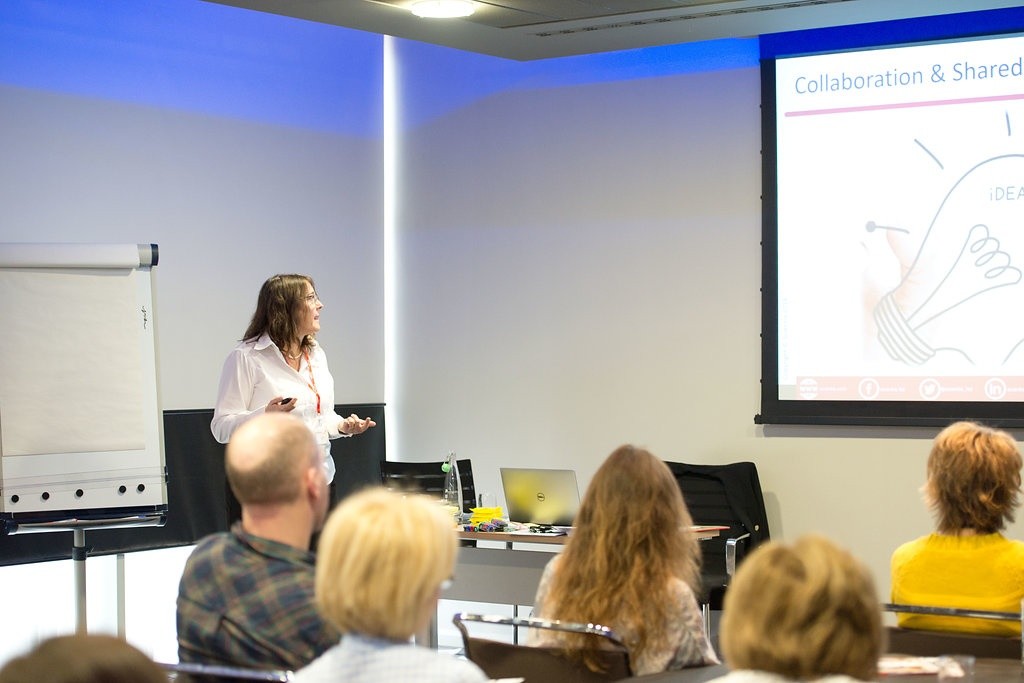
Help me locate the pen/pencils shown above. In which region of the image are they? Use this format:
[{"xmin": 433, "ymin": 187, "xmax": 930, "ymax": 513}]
[{"xmin": 479, "ymin": 494, "xmax": 482, "ymax": 507}]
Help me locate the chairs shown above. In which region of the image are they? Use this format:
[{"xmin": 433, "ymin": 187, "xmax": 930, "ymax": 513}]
[
  {"xmin": 454, "ymin": 613, "xmax": 636, "ymax": 683},
  {"xmin": 881, "ymin": 603, "xmax": 1024, "ymax": 683},
  {"xmin": 665, "ymin": 460, "xmax": 768, "ymax": 646}
]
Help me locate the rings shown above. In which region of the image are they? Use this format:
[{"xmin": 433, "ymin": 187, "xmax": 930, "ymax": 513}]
[{"xmin": 348, "ymin": 426, "xmax": 355, "ymax": 429}]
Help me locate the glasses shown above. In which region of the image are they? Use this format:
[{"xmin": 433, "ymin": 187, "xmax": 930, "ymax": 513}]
[{"xmin": 440, "ymin": 578, "xmax": 454, "ymax": 590}]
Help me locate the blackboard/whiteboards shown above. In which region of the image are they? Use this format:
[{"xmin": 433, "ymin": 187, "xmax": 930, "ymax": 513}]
[{"xmin": 0, "ymin": 266, "xmax": 169, "ymax": 524}]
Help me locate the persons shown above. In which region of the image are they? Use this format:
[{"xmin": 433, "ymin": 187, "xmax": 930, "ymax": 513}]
[
  {"xmin": 210, "ymin": 274, "xmax": 376, "ymax": 551},
  {"xmin": 176, "ymin": 411, "xmax": 342, "ymax": 672},
  {"xmin": 287, "ymin": 487, "xmax": 489, "ymax": 683},
  {"xmin": 705, "ymin": 534, "xmax": 888, "ymax": 683},
  {"xmin": 529, "ymin": 444, "xmax": 721, "ymax": 677},
  {"xmin": 891, "ymin": 421, "xmax": 1024, "ymax": 637},
  {"xmin": 0, "ymin": 633, "xmax": 169, "ymax": 683}
]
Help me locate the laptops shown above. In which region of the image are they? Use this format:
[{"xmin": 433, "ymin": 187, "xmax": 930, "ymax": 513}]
[{"xmin": 500, "ymin": 468, "xmax": 583, "ymax": 526}]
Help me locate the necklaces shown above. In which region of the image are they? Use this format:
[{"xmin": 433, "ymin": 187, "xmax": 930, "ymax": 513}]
[{"xmin": 284, "ymin": 351, "xmax": 303, "ymax": 359}]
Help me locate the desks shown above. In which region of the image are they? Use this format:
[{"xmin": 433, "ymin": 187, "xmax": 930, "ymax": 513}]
[
  {"xmin": 437, "ymin": 597, "xmax": 534, "ymax": 647},
  {"xmin": 620, "ymin": 650, "xmax": 1024, "ymax": 683},
  {"xmin": 454, "ymin": 523, "xmax": 732, "ymax": 555}
]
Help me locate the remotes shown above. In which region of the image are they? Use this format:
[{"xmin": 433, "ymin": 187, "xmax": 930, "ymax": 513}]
[{"xmin": 281, "ymin": 398, "xmax": 292, "ymax": 406}]
